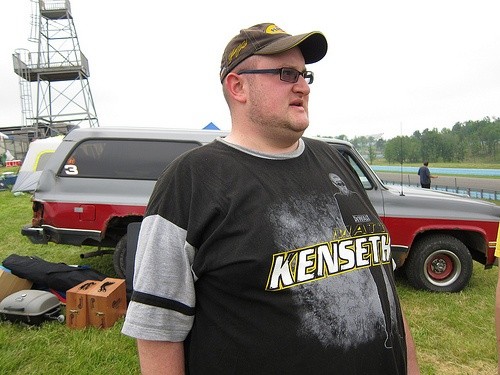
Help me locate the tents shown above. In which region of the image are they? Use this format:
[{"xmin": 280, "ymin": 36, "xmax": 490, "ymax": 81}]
[{"xmin": 10, "ymin": 135, "xmax": 66, "ymax": 192}]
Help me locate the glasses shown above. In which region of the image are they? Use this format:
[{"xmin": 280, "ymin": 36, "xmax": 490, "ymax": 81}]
[{"xmin": 235, "ymin": 67, "xmax": 315, "ymax": 87}]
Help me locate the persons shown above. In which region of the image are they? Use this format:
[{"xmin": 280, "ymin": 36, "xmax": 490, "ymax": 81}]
[
  {"xmin": 417, "ymin": 161, "xmax": 439, "ymax": 189},
  {"xmin": 119, "ymin": 23, "xmax": 420, "ymax": 375}
]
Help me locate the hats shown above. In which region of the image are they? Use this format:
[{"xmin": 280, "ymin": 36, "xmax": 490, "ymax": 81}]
[{"xmin": 219, "ymin": 22, "xmax": 328, "ymax": 85}]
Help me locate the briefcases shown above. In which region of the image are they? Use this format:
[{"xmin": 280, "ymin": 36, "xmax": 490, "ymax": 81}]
[
  {"xmin": 86, "ymin": 277, "xmax": 126, "ymax": 330},
  {"xmin": 66, "ymin": 279, "xmax": 101, "ymax": 331},
  {"xmin": 1, "ymin": 289, "xmax": 62, "ymax": 327}
]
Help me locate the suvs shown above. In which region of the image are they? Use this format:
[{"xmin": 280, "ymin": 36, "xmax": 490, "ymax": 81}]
[{"xmin": 22, "ymin": 129, "xmax": 500, "ymax": 293}]
